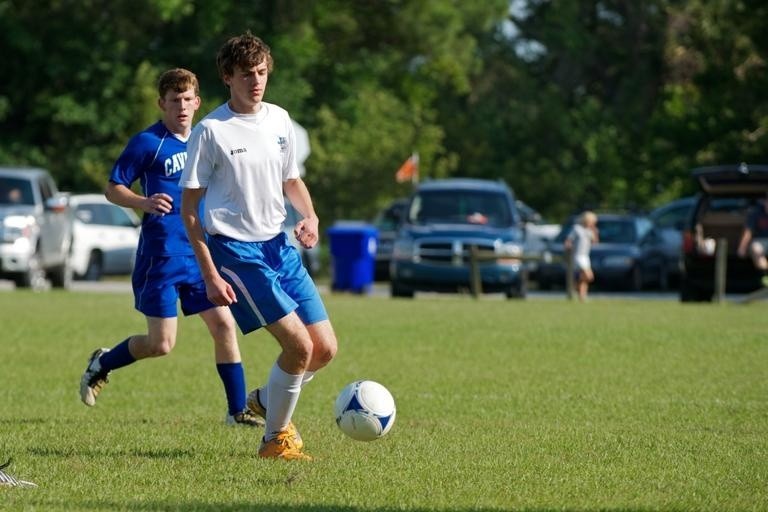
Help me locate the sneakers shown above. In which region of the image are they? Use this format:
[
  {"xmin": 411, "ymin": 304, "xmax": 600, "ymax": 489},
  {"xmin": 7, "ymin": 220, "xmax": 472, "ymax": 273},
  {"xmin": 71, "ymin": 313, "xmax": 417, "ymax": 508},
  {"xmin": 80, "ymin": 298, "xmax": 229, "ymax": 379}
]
[
  {"xmin": 225, "ymin": 407, "xmax": 266, "ymax": 428},
  {"xmin": 258, "ymin": 431, "xmax": 311, "ymax": 460},
  {"xmin": 247, "ymin": 389, "xmax": 303, "ymax": 449},
  {"xmin": 80, "ymin": 348, "xmax": 109, "ymax": 407}
]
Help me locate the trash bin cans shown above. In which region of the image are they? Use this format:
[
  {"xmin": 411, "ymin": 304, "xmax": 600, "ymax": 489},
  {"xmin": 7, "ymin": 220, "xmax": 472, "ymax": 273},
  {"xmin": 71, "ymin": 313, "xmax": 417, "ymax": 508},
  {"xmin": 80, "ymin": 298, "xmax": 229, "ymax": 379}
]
[{"xmin": 325, "ymin": 219, "xmax": 378, "ymax": 294}]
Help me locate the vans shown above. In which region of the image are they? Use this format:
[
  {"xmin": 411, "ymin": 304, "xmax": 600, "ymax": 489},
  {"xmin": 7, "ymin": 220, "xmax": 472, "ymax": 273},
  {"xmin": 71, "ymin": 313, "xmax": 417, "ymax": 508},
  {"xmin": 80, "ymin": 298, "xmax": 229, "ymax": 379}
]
[
  {"xmin": 514, "ymin": 199, "xmax": 564, "ymax": 268},
  {"xmin": 681, "ymin": 163, "xmax": 767, "ymax": 303},
  {"xmin": 634, "ymin": 198, "xmax": 743, "ymax": 289}
]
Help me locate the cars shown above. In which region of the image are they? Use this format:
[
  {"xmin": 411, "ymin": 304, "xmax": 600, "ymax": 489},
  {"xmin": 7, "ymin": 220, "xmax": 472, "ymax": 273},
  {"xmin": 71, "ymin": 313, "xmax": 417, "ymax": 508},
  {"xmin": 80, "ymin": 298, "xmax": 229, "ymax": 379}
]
[
  {"xmin": 371, "ymin": 205, "xmax": 411, "ymax": 261},
  {"xmin": 538, "ymin": 213, "xmax": 654, "ymax": 290},
  {"xmin": 283, "ymin": 196, "xmax": 321, "ymax": 277},
  {"xmin": 1, "ymin": 167, "xmax": 73, "ymax": 291}
]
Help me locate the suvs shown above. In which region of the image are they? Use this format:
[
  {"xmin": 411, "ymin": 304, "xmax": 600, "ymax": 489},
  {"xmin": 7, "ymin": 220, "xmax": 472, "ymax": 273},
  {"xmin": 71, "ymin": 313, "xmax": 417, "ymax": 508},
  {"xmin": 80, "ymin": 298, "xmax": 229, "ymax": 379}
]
[
  {"xmin": 389, "ymin": 179, "xmax": 527, "ymax": 299},
  {"xmin": 69, "ymin": 193, "xmax": 142, "ymax": 280}
]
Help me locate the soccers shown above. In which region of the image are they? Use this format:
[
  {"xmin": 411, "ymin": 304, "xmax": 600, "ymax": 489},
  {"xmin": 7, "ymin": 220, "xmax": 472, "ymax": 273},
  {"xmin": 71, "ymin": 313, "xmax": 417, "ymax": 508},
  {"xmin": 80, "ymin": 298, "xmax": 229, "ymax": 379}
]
[{"xmin": 336, "ymin": 381, "xmax": 397, "ymax": 441}]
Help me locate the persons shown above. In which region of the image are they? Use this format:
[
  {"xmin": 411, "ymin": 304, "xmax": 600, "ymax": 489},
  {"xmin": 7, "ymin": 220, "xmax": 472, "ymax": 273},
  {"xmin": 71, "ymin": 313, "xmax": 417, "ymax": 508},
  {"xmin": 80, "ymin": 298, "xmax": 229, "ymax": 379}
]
[
  {"xmin": 180, "ymin": 34, "xmax": 338, "ymax": 460},
  {"xmin": 736, "ymin": 193, "xmax": 768, "ymax": 271},
  {"xmin": 79, "ymin": 70, "xmax": 267, "ymax": 428},
  {"xmin": 565, "ymin": 213, "xmax": 597, "ymax": 304}
]
